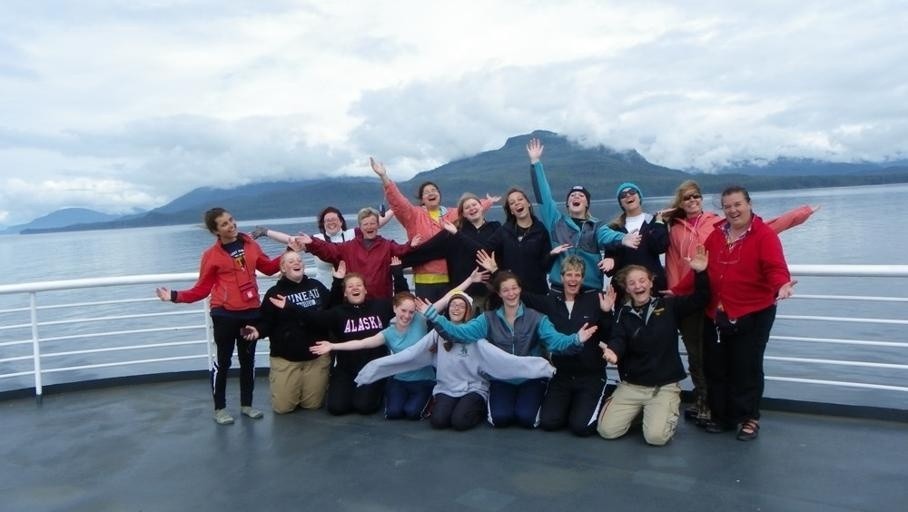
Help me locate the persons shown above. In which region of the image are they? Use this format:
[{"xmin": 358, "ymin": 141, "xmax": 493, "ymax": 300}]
[
  {"xmin": 154, "ymin": 206, "xmax": 303, "ymax": 424},
  {"xmin": 663, "ymin": 178, "xmax": 821, "ymax": 427},
  {"xmin": 241, "ymin": 137, "xmax": 689, "ymax": 446},
  {"xmin": 658, "ymin": 185, "xmax": 799, "ymax": 441}
]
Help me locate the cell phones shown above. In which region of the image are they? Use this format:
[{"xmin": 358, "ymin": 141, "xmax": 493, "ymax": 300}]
[{"xmin": 240, "ymin": 327, "xmax": 251, "ymax": 335}]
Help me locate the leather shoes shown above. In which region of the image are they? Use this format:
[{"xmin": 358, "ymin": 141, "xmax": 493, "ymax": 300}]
[
  {"xmin": 705, "ymin": 421, "xmax": 734, "ymax": 433},
  {"xmin": 737, "ymin": 421, "xmax": 759, "ymax": 441}
]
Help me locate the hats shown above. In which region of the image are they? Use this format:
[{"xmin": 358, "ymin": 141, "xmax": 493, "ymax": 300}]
[
  {"xmin": 567, "ymin": 185, "xmax": 591, "ymax": 204},
  {"xmin": 617, "ymin": 183, "xmax": 643, "ymax": 205}
]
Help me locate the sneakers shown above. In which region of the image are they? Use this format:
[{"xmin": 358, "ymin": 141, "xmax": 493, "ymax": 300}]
[
  {"xmin": 240, "ymin": 406, "xmax": 264, "ymax": 419},
  {"xmin": 684, "ymin": 394, "xmax": 710, "ymax": 427},
  {"xmin": 212, "ymin": 409, "xmax": 235, "ymax": 424}
]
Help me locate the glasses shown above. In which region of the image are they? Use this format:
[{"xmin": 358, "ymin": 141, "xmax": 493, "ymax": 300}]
[
  {"xmin": 683, "ymin": 193, "xmax": 700, "ymax": 200},
  {"xmin": 619, "ymin": 189, "xmax": 637, "ymax": 198}
]
